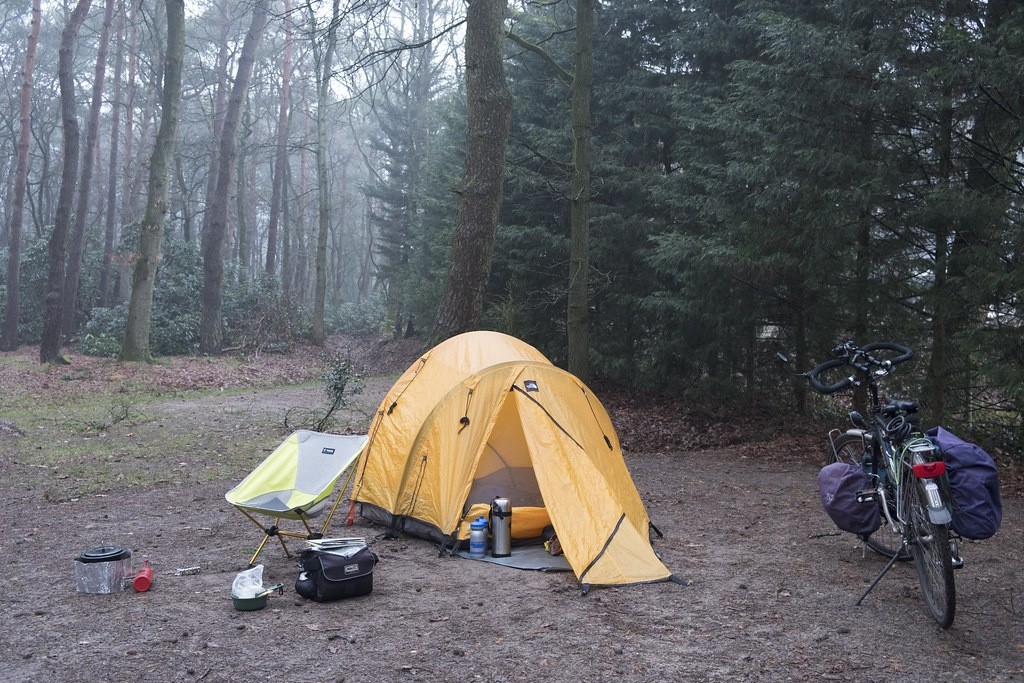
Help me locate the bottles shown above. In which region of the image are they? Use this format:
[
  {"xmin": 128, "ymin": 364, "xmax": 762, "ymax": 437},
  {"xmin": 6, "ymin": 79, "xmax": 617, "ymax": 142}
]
[
  {"xmin": 470, "ymin": 518, "xmax": 486, "ymax": 559},
  {"xmin": 475, "ymin": 515, "xmax": 489, "ymax": 555},
  {"xmin": 134, "ymin": 559, "xmax": 153, "ymax": 592}
]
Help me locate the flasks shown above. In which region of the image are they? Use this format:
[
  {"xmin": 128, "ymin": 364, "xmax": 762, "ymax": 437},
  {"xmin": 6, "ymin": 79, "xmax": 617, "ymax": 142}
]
[{"xmin": 489, "ymin": 496, "xmax": 513, "ymax": 558}]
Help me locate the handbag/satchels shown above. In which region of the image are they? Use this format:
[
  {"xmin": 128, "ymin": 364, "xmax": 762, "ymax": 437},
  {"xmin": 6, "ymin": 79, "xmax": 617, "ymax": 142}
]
[{"xmin": 296, "ymin": 545, "xmax": 378, "ymax": 602}]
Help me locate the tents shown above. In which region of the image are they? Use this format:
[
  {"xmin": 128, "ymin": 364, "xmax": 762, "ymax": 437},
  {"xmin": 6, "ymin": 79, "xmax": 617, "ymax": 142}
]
[{"xmin": 346, "ymin": 330, "xmax": 688, "ymax": 595}]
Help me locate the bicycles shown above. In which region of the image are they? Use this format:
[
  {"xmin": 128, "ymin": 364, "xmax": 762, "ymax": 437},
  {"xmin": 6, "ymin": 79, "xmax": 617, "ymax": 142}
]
[{"xmin": 775, "ymin": 341, "xmax": 965, "ymax": 630}]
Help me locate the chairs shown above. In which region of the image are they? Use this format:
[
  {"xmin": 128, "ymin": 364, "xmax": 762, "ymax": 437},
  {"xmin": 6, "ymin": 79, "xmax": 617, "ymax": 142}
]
[{"xmin": 224, "ymin": 429, "xmax": 371, "ymax": 573}]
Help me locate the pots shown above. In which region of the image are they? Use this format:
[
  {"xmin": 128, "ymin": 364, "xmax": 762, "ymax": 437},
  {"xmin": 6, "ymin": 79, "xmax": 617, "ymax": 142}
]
[{"xmin": 72, "ymin": 540, "xmax": 132, "ymax": 594}]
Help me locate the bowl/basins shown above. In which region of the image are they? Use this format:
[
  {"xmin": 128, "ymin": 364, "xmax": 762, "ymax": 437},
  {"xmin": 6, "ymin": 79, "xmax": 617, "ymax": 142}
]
[{"xmin": 231, "ymin": 588, "xmax": 267, "ymax": 611}]
